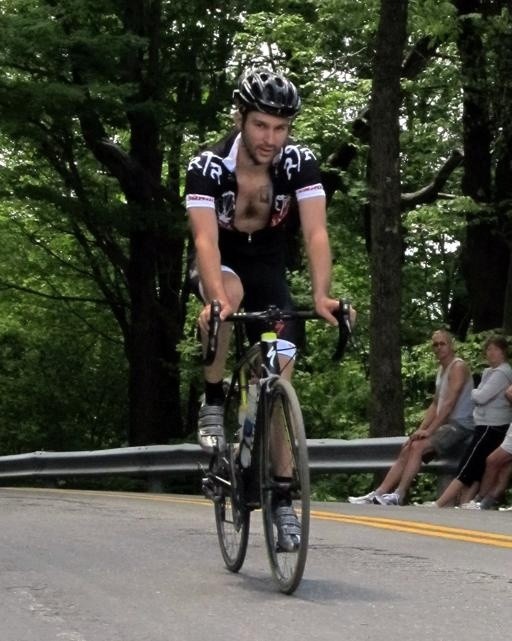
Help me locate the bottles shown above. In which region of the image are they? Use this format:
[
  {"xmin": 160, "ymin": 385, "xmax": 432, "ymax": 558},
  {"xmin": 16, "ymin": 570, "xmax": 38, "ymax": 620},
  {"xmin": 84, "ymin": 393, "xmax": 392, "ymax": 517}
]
[{"xmin": 238, "ymin": 383, "xmax": 260, "ymax": 468}]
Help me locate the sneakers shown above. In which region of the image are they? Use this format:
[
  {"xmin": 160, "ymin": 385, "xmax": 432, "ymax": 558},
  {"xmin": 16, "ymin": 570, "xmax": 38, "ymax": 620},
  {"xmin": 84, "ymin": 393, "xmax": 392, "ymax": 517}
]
[
  {"xmin": 268, "ymin": 501, "xmax": 303, "ymax": 552},
  {"xmin": 199, "ymin": 398, "xmax": 227, "ymax": 456},
  {"xmin": 346, "ymin": 493, "xmax": 497, "ymax": 511}
]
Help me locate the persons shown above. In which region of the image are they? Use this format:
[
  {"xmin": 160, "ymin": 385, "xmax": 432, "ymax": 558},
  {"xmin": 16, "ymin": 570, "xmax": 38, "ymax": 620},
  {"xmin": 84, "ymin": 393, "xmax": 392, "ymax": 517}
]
[
  {"xmin": 456, "ymin": 385, "xmax": 512, "ymax": 509},
  {"xmin": 412, "ymin": 336, "xmax": 512, "ymax": 505},
  {"xmin": 183, "ymin": 65, "xmax": 357, "ymax": 550},
  {"xmin": 347, "ymin": 327, "xmax": 475, "ymax": 503}
]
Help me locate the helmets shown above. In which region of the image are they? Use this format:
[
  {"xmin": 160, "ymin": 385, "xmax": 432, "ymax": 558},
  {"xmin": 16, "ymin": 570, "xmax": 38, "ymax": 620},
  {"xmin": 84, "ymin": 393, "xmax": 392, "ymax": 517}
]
[{"xmin": 234, "ymin": 70, "xmax": 301, "ymax": 119}]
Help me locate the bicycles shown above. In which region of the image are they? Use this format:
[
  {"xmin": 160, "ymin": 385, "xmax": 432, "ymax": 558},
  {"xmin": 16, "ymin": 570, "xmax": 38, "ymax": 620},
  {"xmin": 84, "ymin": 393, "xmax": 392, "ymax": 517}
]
[{"xmin": 202, "ymin": 299, "xmax": 354, "ymax": 593}]
